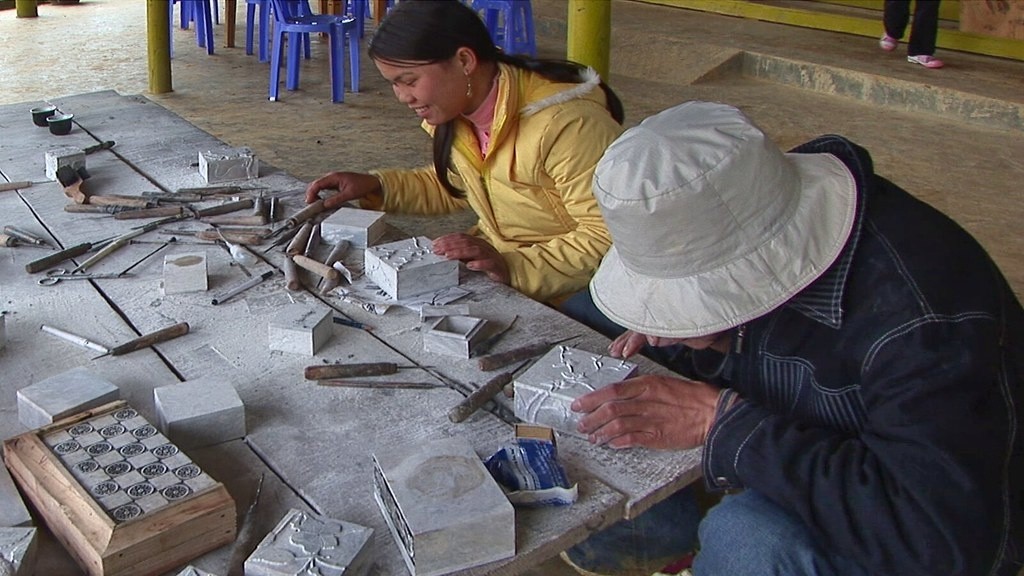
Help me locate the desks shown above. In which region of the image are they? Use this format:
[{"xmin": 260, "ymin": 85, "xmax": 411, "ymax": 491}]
[{"xmin": 1, "ymin": 89, "xmax": 703, "ymax": 576}]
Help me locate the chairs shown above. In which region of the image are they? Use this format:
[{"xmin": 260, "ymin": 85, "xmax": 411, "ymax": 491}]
[{"xmin": 170, "ymin": 0, "xmax": 537, "ymax": 103}]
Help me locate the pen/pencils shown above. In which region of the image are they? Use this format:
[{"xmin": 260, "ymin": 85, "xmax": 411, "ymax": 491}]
[
  {"xmin": 212, "ymin": 267, "xmax": 275, "ymax": 306},
  {"xmin": 334, "ymin": 315, "xmax": 373, "ymax": 330}
]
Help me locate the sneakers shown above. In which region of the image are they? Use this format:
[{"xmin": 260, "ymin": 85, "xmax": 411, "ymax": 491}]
[
  {"xmin": 879, "ymin": 31, "xmax": 899, "ymax": 51},
  {"xmin": 907, "ymin": 54, "xmax": 944, "ymax": 67}
]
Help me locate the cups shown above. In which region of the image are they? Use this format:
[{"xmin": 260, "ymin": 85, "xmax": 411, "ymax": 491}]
[
  {"xmin": 30, "ymin": 105, "xmax": 57, "ymax": 127},
  {"xmin": 47, "ymin": 113, "xmax": 74, "ymax": 135}
]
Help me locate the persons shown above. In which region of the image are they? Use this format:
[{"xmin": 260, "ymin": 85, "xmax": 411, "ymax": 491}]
[
  {"xmin": 569, "ymin": 101, "xmax": 1024, "ymax": 576},
  {"xmin": 306, "ymin": 0, "xmax": 627, "ymax": 302},
  {"xmin": 880, "ymin": 0, "xmax": 944, "ymax": 68}
]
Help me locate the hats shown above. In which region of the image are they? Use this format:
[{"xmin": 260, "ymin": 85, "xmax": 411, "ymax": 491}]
[{"xmin": 588, "ymin": 99, "xmax": 857, "ymax": 338}]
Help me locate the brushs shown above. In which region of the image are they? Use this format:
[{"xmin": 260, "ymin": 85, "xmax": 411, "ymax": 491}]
[{"xmin": 55, "ymin": 166, "xmax": 91, "ymax": 205}]
